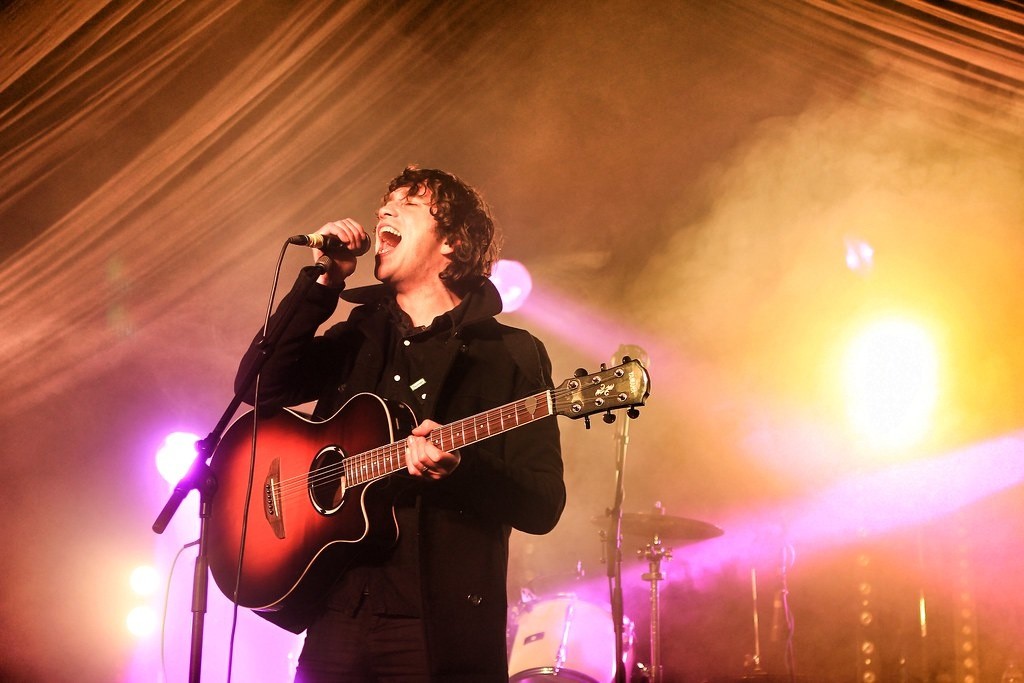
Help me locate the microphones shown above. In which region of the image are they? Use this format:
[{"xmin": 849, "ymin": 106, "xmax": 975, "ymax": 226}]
[
  {"xmin": 770, "ymin": 581, "xmax": 785, "ymax": 642},
  {"xmin": 290, "ymin": 231, "xmax": 371, "ymax": 256}
]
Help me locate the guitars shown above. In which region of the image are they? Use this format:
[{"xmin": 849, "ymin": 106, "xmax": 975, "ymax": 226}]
[{"xmin": 206, "ymin": 355, "xmax": 651, "ymax": 612}]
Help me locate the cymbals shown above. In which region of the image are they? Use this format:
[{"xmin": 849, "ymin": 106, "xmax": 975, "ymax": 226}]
[{"xmin": 589, "ymin": 512, "xmax": 724, "ymax": 544}]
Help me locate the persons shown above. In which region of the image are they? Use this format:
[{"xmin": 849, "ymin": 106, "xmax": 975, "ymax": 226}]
[{"xmin": 235, "ymin": 160, "xmax": 568, "ymax": 683}]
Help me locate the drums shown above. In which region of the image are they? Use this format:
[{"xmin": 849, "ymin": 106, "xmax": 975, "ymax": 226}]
[{"xmin": 508, "ymin": 590, "xmax": 636, "ymax": 683}]
